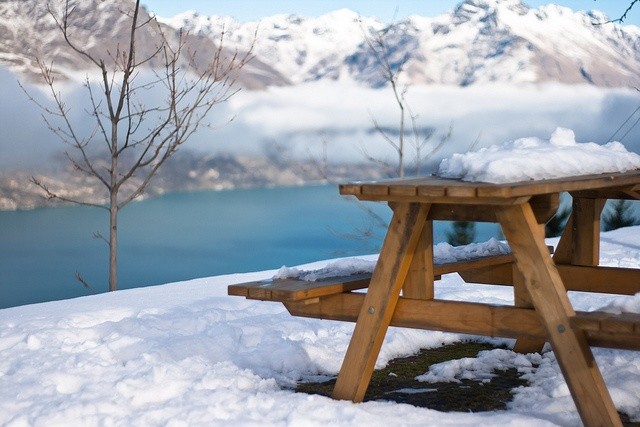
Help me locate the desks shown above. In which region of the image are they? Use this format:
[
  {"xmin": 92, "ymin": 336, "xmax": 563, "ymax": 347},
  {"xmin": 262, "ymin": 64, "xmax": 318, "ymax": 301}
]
[{"xmin": 338, "ymin": 169, "xmax": 639, "ymax": 427}]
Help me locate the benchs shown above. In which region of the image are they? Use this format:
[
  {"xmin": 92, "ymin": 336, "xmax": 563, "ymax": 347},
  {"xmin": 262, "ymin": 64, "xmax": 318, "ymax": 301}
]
[{"xmin": 227, "ymin": 243, "xmax": 559, "ymax": 304}]
